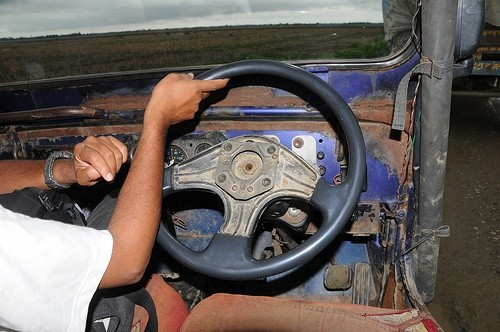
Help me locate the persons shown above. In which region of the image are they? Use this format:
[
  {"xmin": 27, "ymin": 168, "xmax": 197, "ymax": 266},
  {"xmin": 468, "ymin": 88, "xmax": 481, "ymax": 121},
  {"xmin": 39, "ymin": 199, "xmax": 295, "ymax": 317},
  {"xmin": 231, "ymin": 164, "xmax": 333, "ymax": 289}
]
[{"xmin": 0, "ymin": 72, "xmax": 230, "ymax": 332}]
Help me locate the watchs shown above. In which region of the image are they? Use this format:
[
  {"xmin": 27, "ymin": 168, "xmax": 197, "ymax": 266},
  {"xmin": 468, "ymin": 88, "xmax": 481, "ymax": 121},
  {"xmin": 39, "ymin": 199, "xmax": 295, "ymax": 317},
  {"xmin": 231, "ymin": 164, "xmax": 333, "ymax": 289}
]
[{"xmin": 44, "ymin": 151, "xmax": 73, "ymax": 190}]
